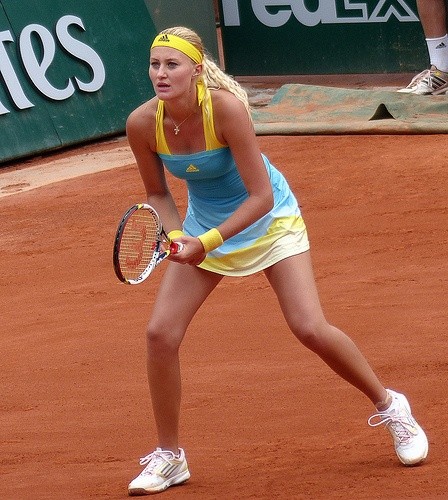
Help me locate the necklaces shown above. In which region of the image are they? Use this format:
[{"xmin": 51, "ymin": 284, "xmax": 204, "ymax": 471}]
[{"xmin": 164, "ymin": 101, "xmax": 194, "ymax": 136}]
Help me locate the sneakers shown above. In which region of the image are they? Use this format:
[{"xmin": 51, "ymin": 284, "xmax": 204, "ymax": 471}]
[
  {"xmin": 377, "ymin": 388, "xmax": 430, "ymax": 465},
  {"xmin": 127, "ymin": 447, "xmax": 190, "ymax": 494},
  {"xmin": 396, "ymin": 65, "xmax": 448, "ymax": 95}
]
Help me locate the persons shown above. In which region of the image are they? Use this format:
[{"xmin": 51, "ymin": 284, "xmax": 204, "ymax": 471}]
[
  {"xmin": 126, "ymin": 26, "xmax": 429, "ymax": 495},
  {"xmin": 395, "ymin": 0, "xmax": 448, "ymax": 96}
]
